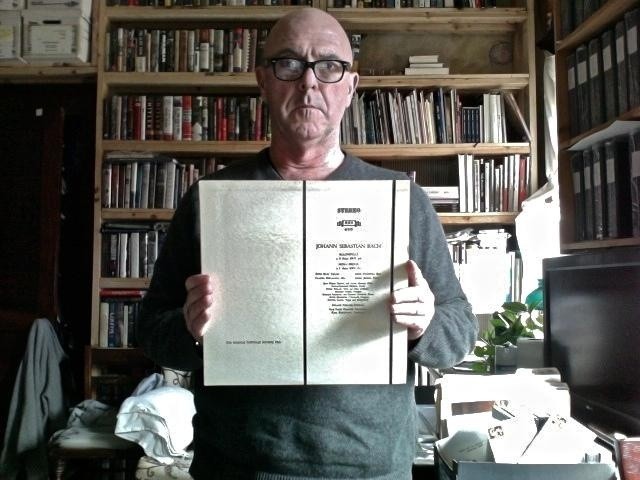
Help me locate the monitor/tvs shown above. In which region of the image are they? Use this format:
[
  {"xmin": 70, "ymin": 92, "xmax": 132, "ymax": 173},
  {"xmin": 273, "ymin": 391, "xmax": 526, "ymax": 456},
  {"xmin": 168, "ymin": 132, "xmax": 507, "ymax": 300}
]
[{"xmin": 542, "ymin": 250, "xmax": 640, "ymax": 436}]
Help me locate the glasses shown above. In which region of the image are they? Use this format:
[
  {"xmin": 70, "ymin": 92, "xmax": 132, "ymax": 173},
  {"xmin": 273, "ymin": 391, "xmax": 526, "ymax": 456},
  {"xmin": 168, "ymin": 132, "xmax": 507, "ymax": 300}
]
[{"xmin": 271, "ymin": 57, "xmax": 351, "ymax": 83}]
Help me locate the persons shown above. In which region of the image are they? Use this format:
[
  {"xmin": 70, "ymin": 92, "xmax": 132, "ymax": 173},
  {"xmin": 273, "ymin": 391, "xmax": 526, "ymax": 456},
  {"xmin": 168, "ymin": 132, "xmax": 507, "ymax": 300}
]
[{"xmin": 136, "ymin": 5, "xmax": 480, "ymax": 480}]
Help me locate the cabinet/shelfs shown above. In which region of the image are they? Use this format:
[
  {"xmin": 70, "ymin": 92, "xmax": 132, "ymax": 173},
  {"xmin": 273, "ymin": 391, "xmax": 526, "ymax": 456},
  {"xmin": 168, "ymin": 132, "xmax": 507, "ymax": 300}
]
[{"xmin": 0, "ymin": 0, "xmax": 640, "ymax": 480}]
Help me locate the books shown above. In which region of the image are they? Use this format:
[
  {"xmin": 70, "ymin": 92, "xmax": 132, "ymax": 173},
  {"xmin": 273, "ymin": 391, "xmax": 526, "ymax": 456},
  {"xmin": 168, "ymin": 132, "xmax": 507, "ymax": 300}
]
[
  {"xmin": 339, "ymin": 33, "xmax": 531, "ymax": 145},
  {"xmin": 102, "ymin": 28, "xmax": 274, "ymax": 141},
  {"xmin": 407, "ymin": 152, "xmax": 531, "ymax": 305},
  {"xmin": 105, "ymin": 1, "xmax": 479, "ymax": 8},
  {"xmin": 99, "ymin": 151, "xmax": 226, "ymax": 349},
  {"xmin": 560, "ymin": 0, "xmax": 640, "ymax": 243}
]
